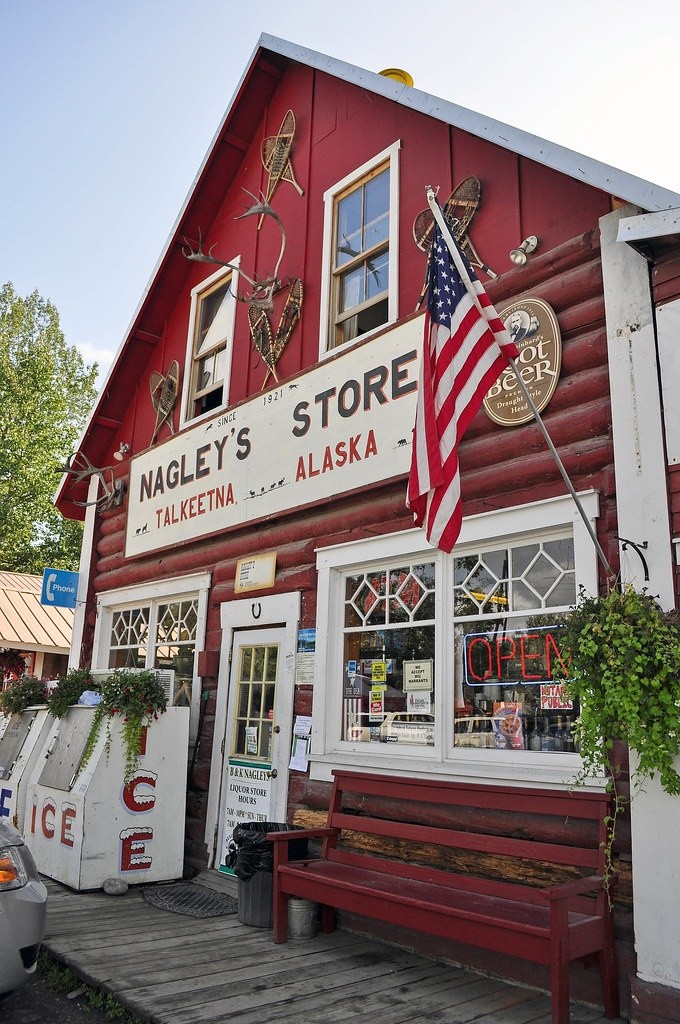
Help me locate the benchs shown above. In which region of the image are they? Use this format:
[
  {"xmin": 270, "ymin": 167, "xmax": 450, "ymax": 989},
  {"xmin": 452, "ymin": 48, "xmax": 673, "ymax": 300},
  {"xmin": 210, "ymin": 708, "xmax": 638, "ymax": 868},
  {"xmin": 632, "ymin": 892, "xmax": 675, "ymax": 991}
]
[{"xmin": 266, "ymin": 769, "xmax": 611, "ymax": 1022}]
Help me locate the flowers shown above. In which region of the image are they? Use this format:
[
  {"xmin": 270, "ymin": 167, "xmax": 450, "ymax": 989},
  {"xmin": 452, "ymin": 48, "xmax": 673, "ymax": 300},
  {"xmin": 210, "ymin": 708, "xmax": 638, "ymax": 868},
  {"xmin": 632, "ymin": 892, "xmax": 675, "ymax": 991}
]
[
  {"xmin": 74, "ymin": 666, "xmax": 167, "ymax": 780},
  {"xmin": 1, "ymin": 674, "xmax": 48, "ymax": 719}
]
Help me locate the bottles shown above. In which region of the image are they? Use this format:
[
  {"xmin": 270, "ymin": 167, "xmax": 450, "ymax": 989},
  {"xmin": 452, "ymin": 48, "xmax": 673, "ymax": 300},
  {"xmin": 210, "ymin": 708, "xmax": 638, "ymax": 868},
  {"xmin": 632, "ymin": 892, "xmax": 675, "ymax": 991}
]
[
  {"xmin": 481, "ymin": 715, "xmax": 491, "ymax": 748},
  {"xmin": 397, "ymin": 716, "xmax": 400, "ymax": 720},
  {"xmin": 413, "ymin": 715, "xmax": 418, "ymax": 722},
  {"xmin": 420, "ymin": 715, "xmax": 425, "ymax": 722},
  {"xmin": 455, "ymin": 714, "xmax": 463, "ymax": 747},
  {"xmin": 473, "ymin": 714, "xmax": 481, "ymax": 748},
  {"xmin": 541, "ymin": 717, "xmax": 554, "ymax": 751},
  {"xmin": 463, "ymin": 714, "xmax": 472, "ymax": 747},
  {"xmin": 529, "ymin": 717, "xmax": 541, "ymax": 750},
  {"xmin": 554, "ymin": 716, "xmax": 563, "ymax": 751},
  {"xmin": 523, "ymin": 717, "xmax": 529, "ymax": 750},
  {"xmin": 563, "ymin": 716, "xmax": 574, "ymax": 751},
  {"xmin": 406, "ymin": 715, "xmax": 409, "ymax": 722},
  {"xmin": 353, "ymin": 722, "xmax": 360, "ymax": 741}
]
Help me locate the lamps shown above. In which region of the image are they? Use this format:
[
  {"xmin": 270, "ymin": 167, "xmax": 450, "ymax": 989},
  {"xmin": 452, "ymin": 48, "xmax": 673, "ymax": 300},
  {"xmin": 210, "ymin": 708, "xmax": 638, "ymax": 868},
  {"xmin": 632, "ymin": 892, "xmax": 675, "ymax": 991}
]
[
  {"xmin": 511, "ymin": 232, "xmax": 540, "ymax": 265},
  {"xmin": 114, "ymin": 442, "xmax": 130, "ymax": 461}
]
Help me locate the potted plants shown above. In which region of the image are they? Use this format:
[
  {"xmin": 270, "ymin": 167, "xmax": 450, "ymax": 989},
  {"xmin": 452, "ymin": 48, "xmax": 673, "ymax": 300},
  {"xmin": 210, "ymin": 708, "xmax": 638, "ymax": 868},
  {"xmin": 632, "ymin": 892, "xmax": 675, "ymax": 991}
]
[{"xmin": 49, "ymin": 668, "xmax": 99, "ymax": 720}]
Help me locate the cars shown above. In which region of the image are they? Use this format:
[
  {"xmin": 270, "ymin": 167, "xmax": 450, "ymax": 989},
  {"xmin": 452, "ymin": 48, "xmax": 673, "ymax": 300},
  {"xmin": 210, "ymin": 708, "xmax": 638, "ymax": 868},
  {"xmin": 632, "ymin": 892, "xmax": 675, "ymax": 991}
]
[{"xmin": 0, "ymin": 814, "xmax": 49, "ymax": 1002}]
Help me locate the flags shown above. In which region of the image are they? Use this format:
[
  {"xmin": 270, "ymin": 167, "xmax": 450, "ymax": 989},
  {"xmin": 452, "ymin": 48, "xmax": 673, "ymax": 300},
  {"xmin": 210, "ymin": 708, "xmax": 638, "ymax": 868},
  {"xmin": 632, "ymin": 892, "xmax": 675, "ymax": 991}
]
[{"xmin": 404, "ymin": 194, "xmax": 521, "ymax": 555}]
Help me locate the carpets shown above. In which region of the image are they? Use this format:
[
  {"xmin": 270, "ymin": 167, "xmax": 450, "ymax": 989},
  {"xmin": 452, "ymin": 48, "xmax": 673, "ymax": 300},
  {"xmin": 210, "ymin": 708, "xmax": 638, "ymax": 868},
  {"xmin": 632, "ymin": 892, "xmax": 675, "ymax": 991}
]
[{"xmin": 143, "ymin": 881, "xmax": 241, "ymax": 919}]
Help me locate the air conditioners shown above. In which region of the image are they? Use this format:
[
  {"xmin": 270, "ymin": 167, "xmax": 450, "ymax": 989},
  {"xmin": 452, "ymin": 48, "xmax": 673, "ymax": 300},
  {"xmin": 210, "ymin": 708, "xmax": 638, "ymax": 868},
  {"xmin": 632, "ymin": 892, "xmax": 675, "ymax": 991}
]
[{"xmin": 89, "ymin": 669, "xmax": 175, "ymax": 705}]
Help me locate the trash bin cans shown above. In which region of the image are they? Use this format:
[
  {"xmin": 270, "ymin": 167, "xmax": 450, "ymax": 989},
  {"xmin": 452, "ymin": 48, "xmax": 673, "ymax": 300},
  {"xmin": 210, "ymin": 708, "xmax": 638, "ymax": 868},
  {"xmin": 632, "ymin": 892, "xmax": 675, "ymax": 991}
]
[
  {"xmin": 233, "ymin": 821, "xmax": 308, "ymax": 929},
  {"xmin": 0, "ymin": 700, "xmax": 191, "ymax": 893}
]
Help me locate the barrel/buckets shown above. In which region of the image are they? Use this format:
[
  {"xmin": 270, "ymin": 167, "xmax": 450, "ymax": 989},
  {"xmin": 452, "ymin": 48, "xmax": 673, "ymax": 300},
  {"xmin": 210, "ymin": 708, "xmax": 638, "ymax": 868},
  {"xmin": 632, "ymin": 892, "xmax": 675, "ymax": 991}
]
[{"xmin": 287, "ymin": 895, "xmax": 316, "ymax": 939}]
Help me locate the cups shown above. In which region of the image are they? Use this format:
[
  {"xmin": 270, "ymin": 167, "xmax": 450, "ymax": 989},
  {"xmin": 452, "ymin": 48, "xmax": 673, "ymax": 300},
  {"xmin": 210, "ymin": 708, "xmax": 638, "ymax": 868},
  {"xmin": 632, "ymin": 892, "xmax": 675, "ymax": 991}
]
[
  {"xmin": 380, "ymin": 722, "xmax": 387, "ymax": 743},
  {"xmin": 500, "ymin": 714, "xmax": 522, "ymax": 749}
]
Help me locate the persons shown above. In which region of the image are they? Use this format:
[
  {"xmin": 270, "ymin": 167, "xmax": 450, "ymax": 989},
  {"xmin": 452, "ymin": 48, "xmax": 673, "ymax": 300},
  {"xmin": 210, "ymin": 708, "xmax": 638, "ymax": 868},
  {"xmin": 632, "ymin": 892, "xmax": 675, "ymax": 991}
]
[{"xmin": 509, "ymin": 313, "xmax": 527, "ymax": 342}]
